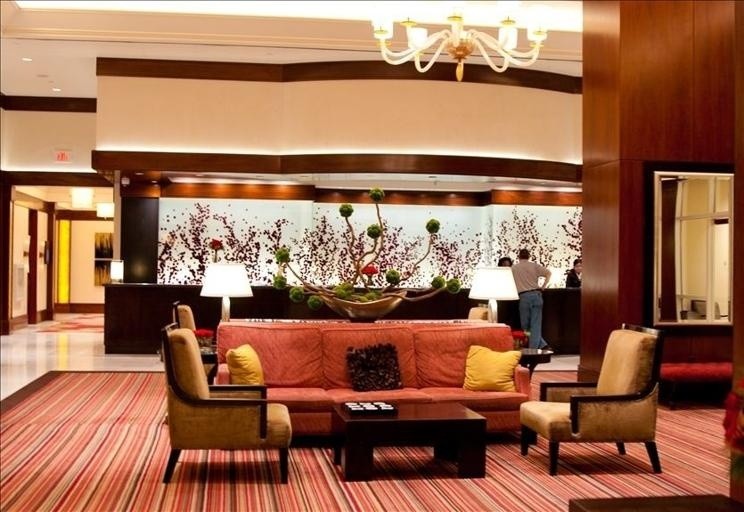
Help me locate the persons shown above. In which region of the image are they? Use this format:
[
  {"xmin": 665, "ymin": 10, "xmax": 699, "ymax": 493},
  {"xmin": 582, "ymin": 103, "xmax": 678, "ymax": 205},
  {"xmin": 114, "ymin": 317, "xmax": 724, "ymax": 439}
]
[
  {"xmin": 498, "ymin": 257, "xmax": 512, "ymax": 266},
  {"xmin": 565, "ymin": 258, "xmax": 582, "ymax": 287},
  {"xmin": 511, "ymin": 249, "xmax": 555, "ymax": 351}
]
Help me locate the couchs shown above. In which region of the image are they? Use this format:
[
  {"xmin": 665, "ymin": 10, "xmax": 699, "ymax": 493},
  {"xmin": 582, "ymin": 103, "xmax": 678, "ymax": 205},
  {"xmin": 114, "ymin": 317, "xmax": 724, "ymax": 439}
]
[{"xmin": 212, "ymin": 318, "xmax": 566, "ymax": 445}]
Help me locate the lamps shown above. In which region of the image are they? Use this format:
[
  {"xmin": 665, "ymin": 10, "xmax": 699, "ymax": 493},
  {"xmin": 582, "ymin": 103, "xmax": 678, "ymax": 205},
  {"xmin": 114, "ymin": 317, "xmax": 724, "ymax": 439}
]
[
  {"xmin": 197, "ymin": 262, "xmax": 255, "ymax": 322},
  {"xmin": 365, "ymin": 1, "xmax": 552, "ymax": 79},
  {"xmin": 467, "ymin": 266, "xmax": 520, "ymax": 319},
  {"xmin": 109, "ymin": 258, "xmax": 123, "ymax": 282},
  {"xmin": 96, "ymin": 203, "xmax": 116, "ymax": 220}
]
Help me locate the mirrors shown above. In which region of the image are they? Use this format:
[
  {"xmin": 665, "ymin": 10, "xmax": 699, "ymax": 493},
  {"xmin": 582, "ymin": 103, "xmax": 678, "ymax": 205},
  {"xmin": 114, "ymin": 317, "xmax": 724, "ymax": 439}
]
[{"xmin": 643, "ymin": 158, "xmax": 733, "ymax": 336}]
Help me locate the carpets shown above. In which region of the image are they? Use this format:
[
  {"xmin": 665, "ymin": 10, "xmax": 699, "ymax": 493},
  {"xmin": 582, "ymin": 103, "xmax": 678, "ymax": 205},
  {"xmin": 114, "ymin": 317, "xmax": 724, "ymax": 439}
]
[{"xmin": 1, "ymin": 366, "xmax": 731, "ymax": 512}]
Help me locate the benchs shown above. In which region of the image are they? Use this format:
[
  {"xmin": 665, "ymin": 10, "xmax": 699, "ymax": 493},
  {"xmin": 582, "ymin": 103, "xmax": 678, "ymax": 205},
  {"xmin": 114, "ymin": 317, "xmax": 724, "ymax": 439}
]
[{"xmin": 653, "ymin": 335, "xmax": 732, "ymax": 410}]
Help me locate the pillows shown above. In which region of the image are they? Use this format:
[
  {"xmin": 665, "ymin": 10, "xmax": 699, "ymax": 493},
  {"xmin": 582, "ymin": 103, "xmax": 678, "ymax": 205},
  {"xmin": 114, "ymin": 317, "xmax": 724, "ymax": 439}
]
[
  {"xmin": 226, "ymin": 344, "xmax": 266, "ymax": 386},
  {"xmin": 344, "ymin": 343, "xmax": 403, "ymax": 392},
  {"xmin": 464, "ymin": 344, "xmax": 521, "ymax": 391}
]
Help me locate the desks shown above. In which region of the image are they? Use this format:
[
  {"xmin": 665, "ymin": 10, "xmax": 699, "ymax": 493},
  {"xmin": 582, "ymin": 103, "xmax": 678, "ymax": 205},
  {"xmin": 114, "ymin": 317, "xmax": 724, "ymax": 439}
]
[{"xmin": 514, "ymin": 345, "xmax": 554, "ymax": 383}]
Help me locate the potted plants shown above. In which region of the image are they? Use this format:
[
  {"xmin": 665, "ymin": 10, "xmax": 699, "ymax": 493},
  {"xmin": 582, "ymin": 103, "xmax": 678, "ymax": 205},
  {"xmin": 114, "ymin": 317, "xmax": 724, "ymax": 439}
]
[{"xmin": 273, "ymin": 185, "xmax": 462, "ymax": 318}]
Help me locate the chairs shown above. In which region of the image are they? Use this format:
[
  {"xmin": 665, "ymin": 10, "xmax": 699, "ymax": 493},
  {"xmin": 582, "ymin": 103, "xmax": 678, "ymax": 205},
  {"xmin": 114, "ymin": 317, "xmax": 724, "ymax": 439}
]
[
  {"xmin": 517, "ymin": 322, "xmax": 664, "ymax": 476},
  {"xmin": 159, "ymin": 322, "xmax": 297, "ymax": 483},
  {"xmin": 167, "ymin": 300, "xmax": 218, "ymax": 385},
  {"xmin": 466, "ymin": 307, "xmax": 488, "ymax": 319}
]
